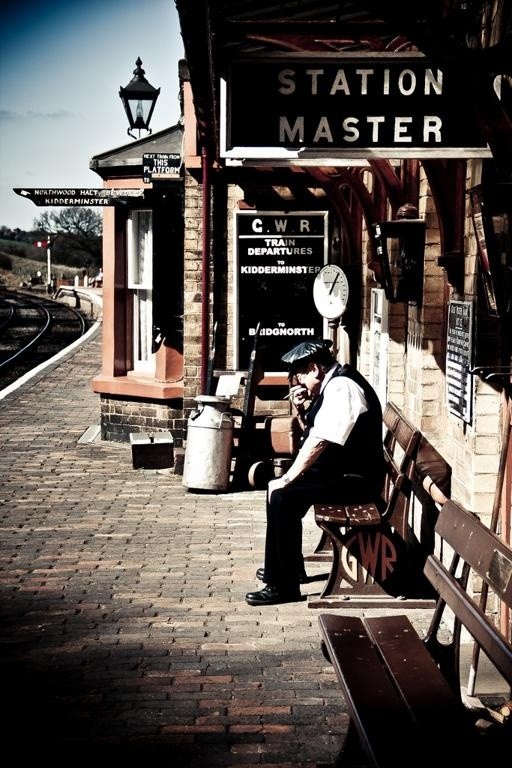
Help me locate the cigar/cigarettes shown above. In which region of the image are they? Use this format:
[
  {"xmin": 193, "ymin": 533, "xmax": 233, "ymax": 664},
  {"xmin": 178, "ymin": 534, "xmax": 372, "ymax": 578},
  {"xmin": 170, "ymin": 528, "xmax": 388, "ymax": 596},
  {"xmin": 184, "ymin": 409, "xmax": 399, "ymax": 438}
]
[{"xmin": 283, "ymin": 392, "xmax": 294, "ymax": 399}]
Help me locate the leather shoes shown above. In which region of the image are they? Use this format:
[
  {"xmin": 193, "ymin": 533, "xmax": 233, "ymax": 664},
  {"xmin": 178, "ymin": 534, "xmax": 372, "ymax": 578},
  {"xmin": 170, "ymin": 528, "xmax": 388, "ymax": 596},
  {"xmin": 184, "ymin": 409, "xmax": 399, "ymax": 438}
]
[
  {"xmin": 246, "ymin": 585, "xmax": 301, "ymax": 605},
  {"xmin": 257, "ymin": 568, "xmax": 308, "ymax": 583}
]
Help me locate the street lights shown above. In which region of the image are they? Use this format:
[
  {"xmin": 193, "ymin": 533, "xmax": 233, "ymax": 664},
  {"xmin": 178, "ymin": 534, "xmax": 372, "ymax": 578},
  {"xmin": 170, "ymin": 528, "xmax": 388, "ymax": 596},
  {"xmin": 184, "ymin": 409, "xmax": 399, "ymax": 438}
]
[{"xmin": 116, "ymin": 54, "xmax": 164, "ymax": 146}]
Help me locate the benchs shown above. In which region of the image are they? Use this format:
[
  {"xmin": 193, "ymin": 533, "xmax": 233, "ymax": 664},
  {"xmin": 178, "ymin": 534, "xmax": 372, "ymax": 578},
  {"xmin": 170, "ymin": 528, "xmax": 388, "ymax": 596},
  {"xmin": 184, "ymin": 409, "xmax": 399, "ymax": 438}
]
[
  {"xmin": 304, "ymin": 402, "xmax": 451, "ymax": 608},
  {"xmin": 307, "ymin": 501, "xmax": 512, "ymax": 765}
]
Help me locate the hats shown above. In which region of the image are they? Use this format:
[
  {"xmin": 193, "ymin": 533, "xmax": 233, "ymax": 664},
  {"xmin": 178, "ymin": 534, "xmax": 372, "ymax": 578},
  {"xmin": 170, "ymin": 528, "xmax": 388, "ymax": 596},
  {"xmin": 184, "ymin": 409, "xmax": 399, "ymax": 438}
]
[{"xmin": 281, "ymin": 340, "xmax": 333, "ymax": 379}]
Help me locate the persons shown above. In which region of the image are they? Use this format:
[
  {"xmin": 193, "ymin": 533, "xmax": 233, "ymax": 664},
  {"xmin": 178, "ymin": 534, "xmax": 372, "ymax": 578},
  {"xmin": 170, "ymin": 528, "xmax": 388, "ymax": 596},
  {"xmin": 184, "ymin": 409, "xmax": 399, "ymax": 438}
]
[
  {"xmin": 88, "ymin": 268, "xmax": 103, "ymax": 287},
  {"xmin": 244, "ymin": 339, "xmax": 386, "ymax": 604}
]
